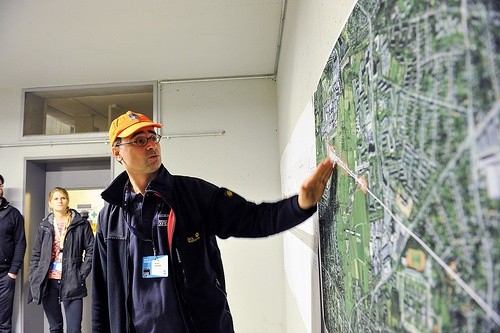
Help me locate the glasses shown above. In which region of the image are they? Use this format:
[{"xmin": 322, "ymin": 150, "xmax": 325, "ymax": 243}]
[{"xmin": 115, "ymin": 134, "xmax": 162, "ymax": 148}]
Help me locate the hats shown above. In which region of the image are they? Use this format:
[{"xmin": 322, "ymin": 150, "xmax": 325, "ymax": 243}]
[{"xmin": 109, "ymin": 110, "xmax": 162, "ymax": 149}]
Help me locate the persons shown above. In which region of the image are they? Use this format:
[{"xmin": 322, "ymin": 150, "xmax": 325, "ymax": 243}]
[
  {"xmin": 26, "ymin": 187, "xmax": 94, "ymax": 333},
  {"xmin": 90, "ymin": 111, "xmax": 334, "ymax": 333},
  {"xmin": 0, "ymin": 175, "xmax": 28, "ymax": 333}
]
